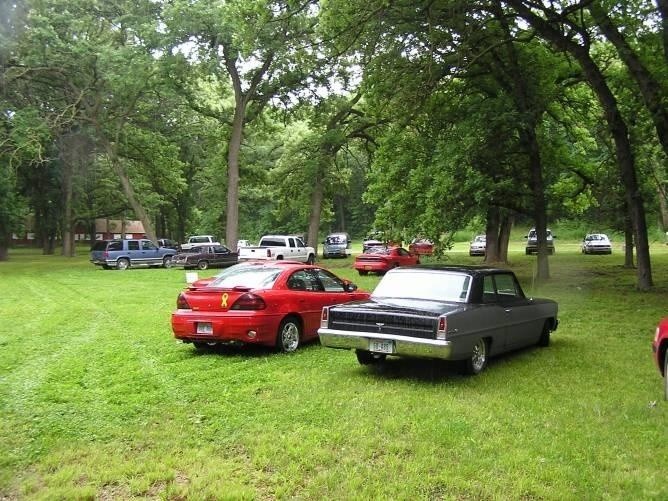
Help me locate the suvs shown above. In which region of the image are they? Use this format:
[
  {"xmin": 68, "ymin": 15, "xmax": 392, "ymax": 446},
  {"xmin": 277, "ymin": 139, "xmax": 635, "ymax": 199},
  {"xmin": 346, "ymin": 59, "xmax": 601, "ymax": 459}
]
[
  {"xmin": 88, "ymin": 239, "xmax": 178, "ymax": 270},
  {"xmin": 362, "ymin": 232, "xmax": 388, "ymax": 251},
  {"xmin": 138, "ymin": 238, "xmax": 178, "ymax": 249},
  {"xmin": 523, "ymin": 228, "xmax": 557, "ymax": 254}
]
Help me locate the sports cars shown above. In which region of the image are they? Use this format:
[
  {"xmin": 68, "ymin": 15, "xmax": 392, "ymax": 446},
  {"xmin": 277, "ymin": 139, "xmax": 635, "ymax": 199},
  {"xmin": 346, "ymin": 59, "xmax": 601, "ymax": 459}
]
[{"xmin": 171, "ymin": 260, "xmax": 371, "ymax": 353}]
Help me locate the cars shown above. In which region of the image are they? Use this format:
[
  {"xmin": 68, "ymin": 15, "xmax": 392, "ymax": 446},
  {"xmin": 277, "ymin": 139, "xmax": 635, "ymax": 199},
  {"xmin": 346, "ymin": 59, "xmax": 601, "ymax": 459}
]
[
  {"xmin": 469, "ymin": 235, "xmax": 487, "ymax": 255},
  {"xmin": 651, "ymin": 314, "xmax": 668, "ymax": 400},
  {"xmin": 172, "ymin": 243, "xmax": 237, "ymax": 269},
  {"xmin": 190, "ymin": 257, "xmax": 307, "ymax": 286},
  {"xmin": 580, "ymin": 234, "xmax": 613, "ymax": 254},
  {"xmin": 317, "ymin": 263, "xmax": 560, "ymax": 374},
  {"xmin": 407, "ymin": 237, "xmax": 434, "ymax": 255},
  {"xmin": 354, "ymin": 247, "xmax": 419, "ymax": 276}
]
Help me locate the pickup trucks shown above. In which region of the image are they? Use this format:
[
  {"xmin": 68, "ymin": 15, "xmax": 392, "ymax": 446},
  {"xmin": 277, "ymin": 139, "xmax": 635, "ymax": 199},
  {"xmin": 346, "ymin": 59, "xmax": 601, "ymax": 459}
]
[
  {"xmin": 180, "ymin": 235, "xmax": 221, "ymax": 250},
  {"xmin": 237, "ymin": 235, "xmax": 316, "ymax": 265}
]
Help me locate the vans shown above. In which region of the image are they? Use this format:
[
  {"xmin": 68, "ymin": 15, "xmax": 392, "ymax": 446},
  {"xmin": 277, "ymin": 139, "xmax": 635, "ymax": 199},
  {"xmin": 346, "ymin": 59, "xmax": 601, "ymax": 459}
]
[{"xmin": 321, "ymin": 232, "xmax": 352, "ymax": 258}]
